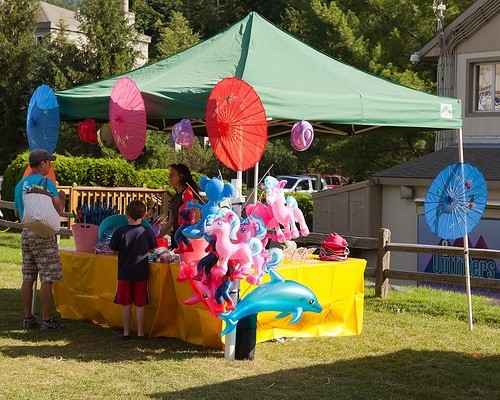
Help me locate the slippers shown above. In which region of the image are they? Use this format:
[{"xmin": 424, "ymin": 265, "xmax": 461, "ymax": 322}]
[
  {"xmin": 118, "ymin": 332, "xmax": 129, "ymax": 340},
  {"xmin": 138, "ymin": 336, "xmax": 149, "ymax": 341}
]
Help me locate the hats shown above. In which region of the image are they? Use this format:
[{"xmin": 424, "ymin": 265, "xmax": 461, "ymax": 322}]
[
  {"xmin": 78, "ymin": 118, "xmax": 97, "ymax": 145},
  {"xmin": 172, "ymin": 119, "xmax": 194, "ymax": 148},
  {"xmin": 291, "ymin": 121, "xmax": 314, "ymax": 151},
  {"xmin": 319, "ymin": 232, "xmax": 349, "ymax": 260},
  {"xmin": 29, "ymin": 149, "xmax": 56, "ymax": 161}
]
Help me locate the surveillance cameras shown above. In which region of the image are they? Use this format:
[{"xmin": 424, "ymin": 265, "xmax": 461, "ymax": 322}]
[{"xmin": 410, "ymin": 55, "xmax": 420, "ymax": 65}]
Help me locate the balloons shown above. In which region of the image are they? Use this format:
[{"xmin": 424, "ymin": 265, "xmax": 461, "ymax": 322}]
[{"xmin": 174, "ymin": 175, "xmax": 324, "ymax": 336}]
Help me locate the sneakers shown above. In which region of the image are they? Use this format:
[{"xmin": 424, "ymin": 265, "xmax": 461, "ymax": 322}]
[
  {"xmin": 23, "ymin": 315, "xmax": 41, "ymax": 329},
  {"xmin": 41, "ymin": 318, "xmax": 66, "ymax": 330}
]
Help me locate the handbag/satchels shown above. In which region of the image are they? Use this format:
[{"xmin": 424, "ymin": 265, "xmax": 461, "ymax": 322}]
[
  {"xmin": 72, "ymin": 223, "xmax": 99, "ymax": 252},
  {"xmin": 21, "ymin": 178, "xmax": 60, "ymax": 238}
]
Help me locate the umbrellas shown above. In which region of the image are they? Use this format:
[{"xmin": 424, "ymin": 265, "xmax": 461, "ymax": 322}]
[
  {"xmin": 205, "ymin": 76, "xmax": 269, "ymax": 172},
  {"xmin": 110, "ymin": 77, "xmax": 146, "ymax": 160},
  {"xmin": 27, "ymin": 83, "xmax": 61, "ymax": 155},
  {"xmin": 423, "ymin": 161, "xmax": 488, "ymax": 240}
]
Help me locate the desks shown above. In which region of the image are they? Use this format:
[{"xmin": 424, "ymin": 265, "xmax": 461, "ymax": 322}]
[{"xmin": 52, "ymin": 248, "xmax": 367, "ymax": 350}]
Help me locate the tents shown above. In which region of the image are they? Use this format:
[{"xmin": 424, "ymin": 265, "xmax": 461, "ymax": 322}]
[{"xmin": 55, "ymin": 10, "xmax": 473, "ymax": 332}]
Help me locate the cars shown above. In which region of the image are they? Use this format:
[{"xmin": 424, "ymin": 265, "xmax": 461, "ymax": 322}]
[
  {"xmin": 275, "ymin": 176, "xmax": 328, "ymax": 193},
  {"xmin": 322, "ymin": 175, "xmax": 349, "ymax": 188}
]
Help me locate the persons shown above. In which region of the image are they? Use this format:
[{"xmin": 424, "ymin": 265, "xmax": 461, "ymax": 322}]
[
  {"xmin": 108, "ymin": 200, "xmax": 158, "ymax": 341},
  {"xmin": 14, "ymin": 149, "xmax": 67, "ymax": 331},
  {"xmin": 158, "ymin": 163, "xmax": 201, "ymax": 253},
  {"xmin": 347, "ymin": 175, "xmax": 356, "ymax": 185}
]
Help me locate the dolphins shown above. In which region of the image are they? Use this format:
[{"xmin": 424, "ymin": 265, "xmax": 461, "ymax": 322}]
[{"xmin": 217, "ymin": 269, "xmax": 323, "ymax": 336}]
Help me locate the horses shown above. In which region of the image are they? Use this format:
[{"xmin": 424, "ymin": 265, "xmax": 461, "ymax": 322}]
[{"xmin": 205, "ymin": 177, "xmax": 310, "ymax": 285}]
